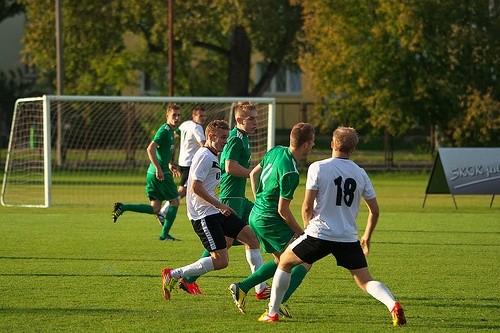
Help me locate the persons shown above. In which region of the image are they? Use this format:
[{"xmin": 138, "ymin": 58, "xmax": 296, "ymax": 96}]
[
  {"xmin": 179, "ymin": 102, "xmax": 271, "ymax": 301},
  {"xmin": 156, "ymin": 107, "xmax": 208, "ymax": 226},
  {"xmin": 163, "ymin": 120, "xmax": 272, "ymax": 301},
  {"xmin": 258, "ymin": 126, "xmax": 406, "ymax": 326},
  {"xmin": 228, "ymin": 122, "xmax": 315, "ymax": 318},
  {"xmin": 112, "ymin": 103, "xmax": 181, "ymax": 242}
]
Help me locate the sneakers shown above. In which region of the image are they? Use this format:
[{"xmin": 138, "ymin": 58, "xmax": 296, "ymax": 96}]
[
  {"xmin": 227, "ymin": 282, "xmax": 247, "ymax": 314},
  {"xmin": 255, "ymin": 286, "xmax": 271, "ymax": 300},
  {"xmin": 390, "ymin": 302, "xmax": 406, "ymax": 325},
  {"xmin": 279, "ymin": 304, "xmax": 293, "ymax": 318},
  {"xmin": 257, "ymin": 311, "xmax": 280, "ymax": 322},
  {"xmin": 156, "ymin": 214, "xmax": 164, "ymax": 227},
  {"xmin": 159, "ymin": 234, "xmax": 178, "ymax": 241},
  {"xmin": 161, "ymin": 267, "xmax": 178, "ymax": 300},
  {"xmin": 178, "ymin": 278, "xmax": 203, "ymax": 295},
  {"xmin": 112, "ymin": 202, "xmax": 124, "ymax": 223}
]
